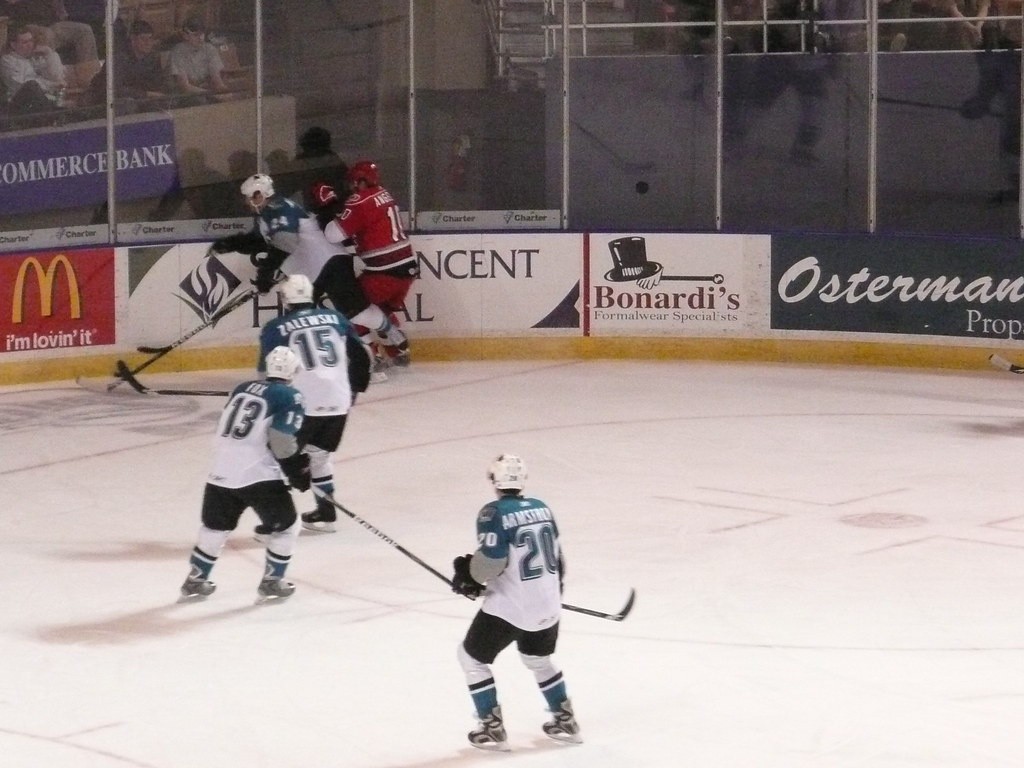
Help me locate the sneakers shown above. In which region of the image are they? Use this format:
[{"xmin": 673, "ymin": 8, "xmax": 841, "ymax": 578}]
[
  {"xmin": 543, "ymin": 699, "xmax": 582, "ymax": 744},
  {"xmin": 468, "ymin": 705, "xmax": 511, "ymax": 751},
  {"xmin": 178, "ymin": 566, "xmax": 216, "ymax": 604},
  {"xmin": 388, "ymin": 324, "xmax": 409, "ymax": 353},
  {"xmin": 388, "ymin": 354, "xmax": 410, "ymax": 371},
  {"xmin": 301, "ymin": 500, "xmax": 336, "ymax": 533},
  {"xmin": 254, "ymin": 561, "xmax": 295, "ymax": 604},
  {"xmin": 368, "ymin": 364, "xmax": 388, "ymax": 383},
  {"xmin": 253, "ymin": 525, "xmax": 271, "ymax": 544}
]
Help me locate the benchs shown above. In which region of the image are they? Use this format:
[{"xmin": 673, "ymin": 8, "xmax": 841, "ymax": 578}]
[{"xmin": 500, "ymin": 0, "xmax": 1023, "ymax": 59}]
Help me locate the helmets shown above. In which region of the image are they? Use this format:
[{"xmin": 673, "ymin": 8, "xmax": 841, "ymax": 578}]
[
  {"xmin": 279, "ymin": 274, "xmax": 313, "ymax": 304},
  {"xmin": 242, "ymin": 173, "xmax": 275, "ymax": 206},
  {"xmin": 265, "ymin": 344, "xmax": 297, "ymax": 386},
  {"xmin": 487, "ymin": 452, "xmax": 528, "ymax": 490},
  {"xmin": 346, "ymin": 161, "xmax": 380, "ymax": 185}
]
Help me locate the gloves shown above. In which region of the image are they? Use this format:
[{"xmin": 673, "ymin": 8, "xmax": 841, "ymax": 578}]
[
  {"xmin": 213, "ymin": 232, "xmax": 242, "ymax": 254},
  {"xmin": 284, "ymin": 452, "xmax": 312, "ymax": 492},
  {"xmin": 350, "ymin": 391, "xmax": 357, "ymax": 406},
  {"xmin": 256, "ymin": 269, "xmax": 274, "ymax": 293},
  {"xmin": 452, "ymin": 554, "xmax": 486, "ymax": 597},
  {"xmin": 313, "ymin": 183, "xmax": 337, "ymax": 207}
]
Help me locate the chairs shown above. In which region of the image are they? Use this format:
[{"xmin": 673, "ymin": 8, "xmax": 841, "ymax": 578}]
[{"xmin": 0, "ymin": 0, "xmax": 262, "ymax": 126}]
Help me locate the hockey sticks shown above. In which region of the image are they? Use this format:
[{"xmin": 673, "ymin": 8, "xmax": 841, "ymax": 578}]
[
  {"xmin": 74, "ymin": 292, "xmax": 256, "ymax": 393},
  {"xmin": 117, "ymin": 359, "xmax": 231, "ymax": 397},
  {"xmin": 308, "ymin": 483, "xmax": 453, "ymax": 585},
  {"xmin": 562, "ymin": 589, "xmax": 637, "ymax": 621}
]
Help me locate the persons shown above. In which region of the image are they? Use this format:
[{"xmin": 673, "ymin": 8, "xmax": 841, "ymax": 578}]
[
  {"xmin": 163, "ymin": 16, "xmax": 232, "ymax": 108},
  {"xmin": 78, "ymin": 20, "xmax": 173, "ymax": 120},
  {"xmin": 658, "ymin": 0, "xmax": 1014, "ymax": 54},
  {"xmin": 963, "ymin": 51, "xmax": 1021, "ymax": 206},
  {"xmin": 726, "ymin": 54, "xmax": 832, "ymax": 169},
  {"xmin": 0, "ymin": 27, "xmax": 66, "ymax": 112},
  {"xmin": 453, "ymin": 455, "xmax": 584, "ymax": 752},
  {"xmin": 13, "ymin": 0, "xmax": 127, "ymax": 64},
  {"xmin": 254, "ymin": 273, "xmax": 370, "ymax": 543},
  {"xmin": 211, "ymin": 173, "xmax": 408, "ymax": 352},
  {"xmin": 324, "ymin": 160, "xmax": 417, "ymax": 384},
  {"xmin": 177, "ymin": 345, "xmax": 311, "ymax": 606},
  {"xmin": 152, "ymin": 127, "xmax": 349, "ymax": 220}
]
